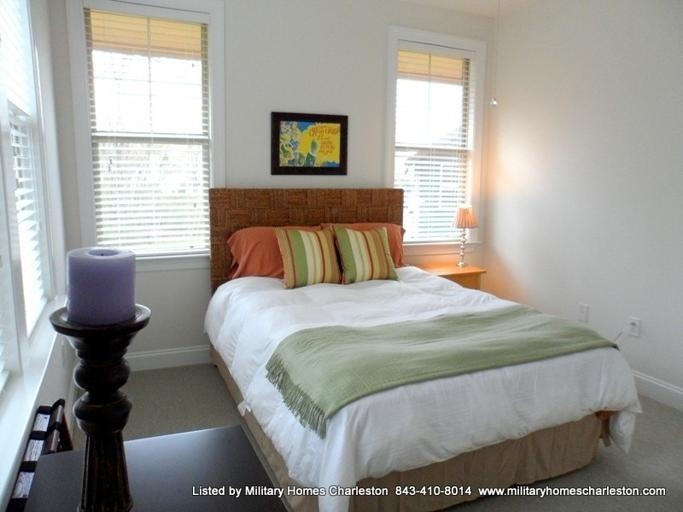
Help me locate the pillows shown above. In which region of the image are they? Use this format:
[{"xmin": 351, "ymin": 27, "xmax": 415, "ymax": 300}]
[{"xmin": 225, "ymin": 222, "xmax": 406, "ymax": 289}]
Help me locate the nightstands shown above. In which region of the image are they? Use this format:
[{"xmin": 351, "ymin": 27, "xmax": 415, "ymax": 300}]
[{"xmin": 414, "ymin": 261, "xmax": 486, "ymax": 291}]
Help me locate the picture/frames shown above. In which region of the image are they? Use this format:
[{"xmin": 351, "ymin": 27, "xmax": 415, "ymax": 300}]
[{"xmin": 270, "ymin": 113, "xmax": 348, "ymax": 176}]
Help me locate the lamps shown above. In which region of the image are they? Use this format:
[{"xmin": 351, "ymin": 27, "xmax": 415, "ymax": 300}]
[{"xmin": 453, "ymin": 205, "xmax": 479, "ymax": 268}]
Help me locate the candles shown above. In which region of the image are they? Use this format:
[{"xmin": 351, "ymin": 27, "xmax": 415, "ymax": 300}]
[{"xmin": 65, "ymin": 246, "xmax": 137, "ymax": 325}]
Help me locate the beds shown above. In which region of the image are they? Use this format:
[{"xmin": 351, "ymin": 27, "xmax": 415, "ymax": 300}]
[{"xmin": 206, "ymin": 186, "xmax": 634, "ymax": 511}]
